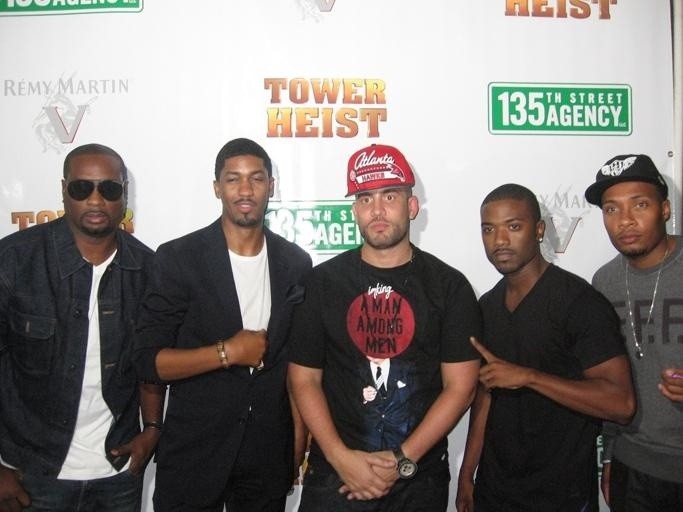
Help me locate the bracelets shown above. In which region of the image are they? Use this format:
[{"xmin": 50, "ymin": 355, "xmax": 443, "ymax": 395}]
[
  {"xmin": 216, "ymin": 339, "xmax": 230, "ymax": 370},
  {"xmin": 143, "ymin": 420, "xmax": 163, "ymax": 433}
]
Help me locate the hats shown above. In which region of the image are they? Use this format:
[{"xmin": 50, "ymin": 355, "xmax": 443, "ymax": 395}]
[
  {"xmin": 583, "ymin": 152, "xmax": 669, "ymax": 209},
  {"xmin": 342, "ymin": 142, "xmax": 417, "ymax": 199}
]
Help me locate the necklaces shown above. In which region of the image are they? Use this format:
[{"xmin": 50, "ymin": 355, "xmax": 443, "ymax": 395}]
[{"xmin": 625, "ymin": 234, "xmax": 671, "ymax": 358}]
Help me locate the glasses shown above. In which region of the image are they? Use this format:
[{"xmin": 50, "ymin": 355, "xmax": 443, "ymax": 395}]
[{"xmin": 66, "ymin": 177, "xmax": 125, "ymax": 203}]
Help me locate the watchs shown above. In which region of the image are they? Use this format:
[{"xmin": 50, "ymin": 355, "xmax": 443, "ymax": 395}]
[{"xmin": 391, "ymin": 447, "xmax": 418, "ymax": 481}]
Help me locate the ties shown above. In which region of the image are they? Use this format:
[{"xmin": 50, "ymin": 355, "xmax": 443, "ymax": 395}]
[{"xmin": 374, "ymin": 366, "xmax": 388, "ymax": 400}]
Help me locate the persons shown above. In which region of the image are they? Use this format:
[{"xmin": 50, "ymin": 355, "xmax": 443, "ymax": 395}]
[
  {"xmin": 0, "ymin": 143, "xmax": 167, "ymax": 512},
  {"xmin": 585, "ymin": 153, "xmax": 683, "ymax": 511},
  {"xmin": 456, "ymin": 183, "xmax": 637, "ymax": 511},
  {"xmin": 287, "ymin": 144, "xmax": 482, "ymax": 511},
  {"xmin": 130, "ymin": 137, "xmax": 312, "ymax": 511}
]
